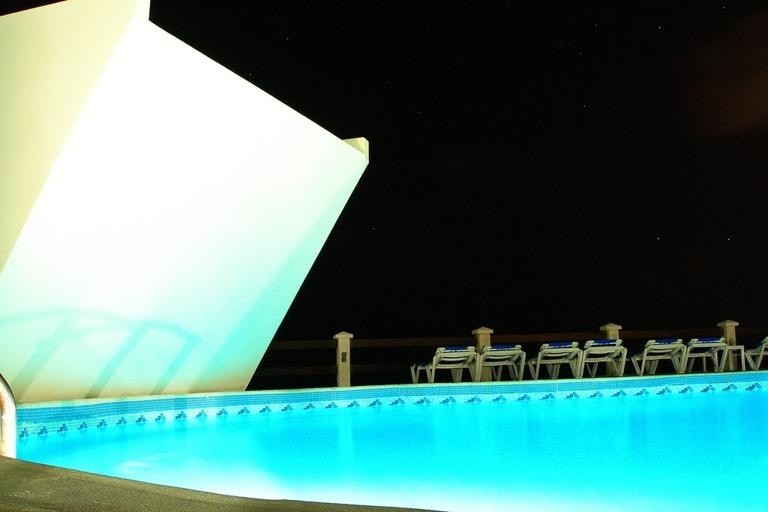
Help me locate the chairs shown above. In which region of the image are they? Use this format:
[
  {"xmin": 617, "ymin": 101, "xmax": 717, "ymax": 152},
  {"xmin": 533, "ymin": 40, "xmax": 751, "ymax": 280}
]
[{"xmin": 411, "ymin": 334, "xmax": 768, "ymax": 383}]
